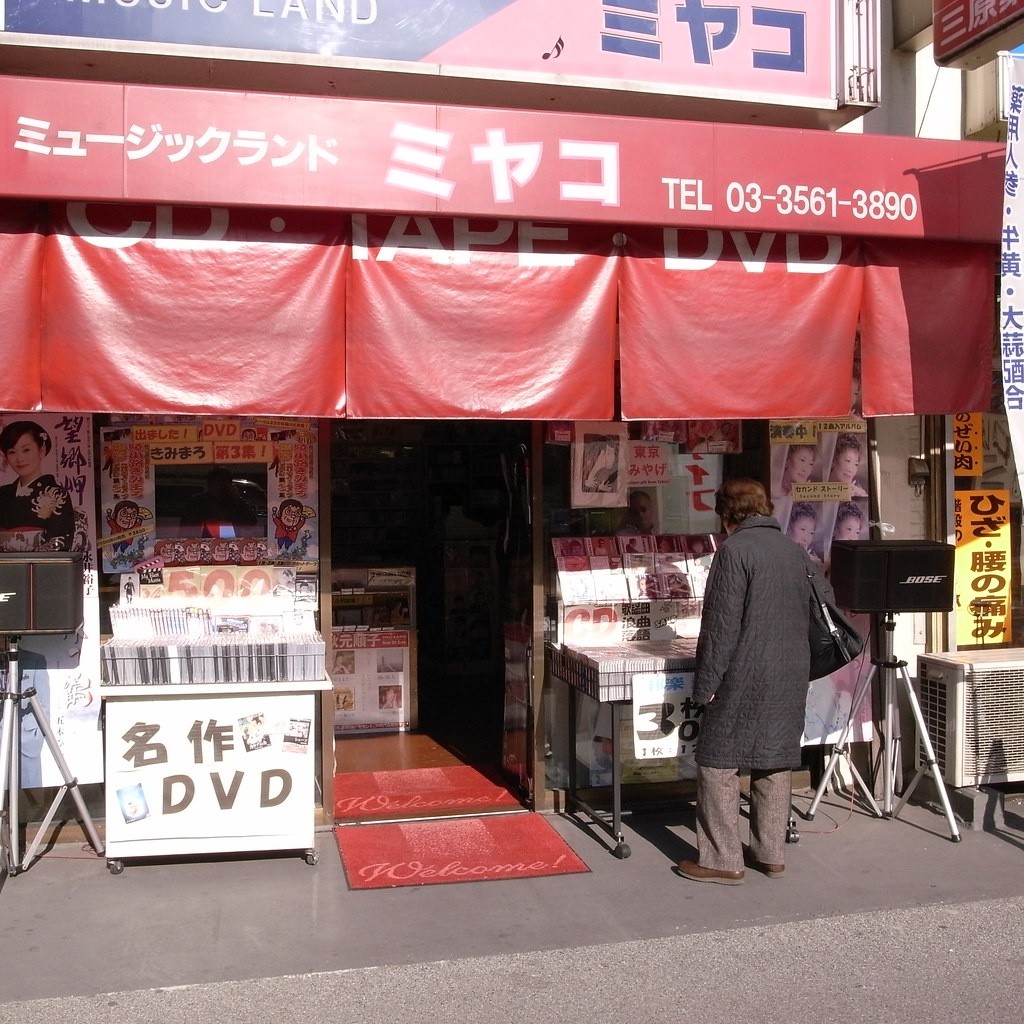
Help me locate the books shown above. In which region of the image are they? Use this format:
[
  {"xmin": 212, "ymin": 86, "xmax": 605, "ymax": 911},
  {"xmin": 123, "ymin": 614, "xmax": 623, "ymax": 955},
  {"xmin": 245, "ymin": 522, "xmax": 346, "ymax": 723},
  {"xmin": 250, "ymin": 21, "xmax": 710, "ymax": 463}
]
[
  {"xmin": 550, "ymin": 534, "xmax": 720, "ymax": 640},
  {"xmin": 101, "ymin": 602, "xmax": 326, "ymax": 684}
]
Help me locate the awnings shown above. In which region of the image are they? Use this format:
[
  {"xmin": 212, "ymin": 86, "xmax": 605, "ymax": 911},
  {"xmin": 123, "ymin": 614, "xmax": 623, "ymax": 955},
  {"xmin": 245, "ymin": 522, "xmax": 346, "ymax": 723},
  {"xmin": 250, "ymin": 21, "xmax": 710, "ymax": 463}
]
[{"xmin": 0, "ymin": 77, "xmax": 1005, "ymax": 421}]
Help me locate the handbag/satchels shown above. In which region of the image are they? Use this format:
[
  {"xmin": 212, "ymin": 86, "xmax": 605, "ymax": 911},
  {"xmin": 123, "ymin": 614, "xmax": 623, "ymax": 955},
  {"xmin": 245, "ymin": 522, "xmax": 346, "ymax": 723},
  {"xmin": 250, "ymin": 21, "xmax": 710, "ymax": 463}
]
[{"xmin": 803, "ymin": 546, "xmax": 865, "ymax": 682}]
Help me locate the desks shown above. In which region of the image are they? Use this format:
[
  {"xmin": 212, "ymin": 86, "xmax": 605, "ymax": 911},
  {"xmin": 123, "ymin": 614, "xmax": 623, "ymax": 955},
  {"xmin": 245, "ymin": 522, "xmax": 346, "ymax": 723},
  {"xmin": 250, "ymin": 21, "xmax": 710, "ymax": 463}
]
[
  {"xmin": 546, "ymin": 635, "xmax": 801, "ymax": 857},
  {"xmin": 98, "ymin": 672, "xmax": 333, "ymax": 873}
]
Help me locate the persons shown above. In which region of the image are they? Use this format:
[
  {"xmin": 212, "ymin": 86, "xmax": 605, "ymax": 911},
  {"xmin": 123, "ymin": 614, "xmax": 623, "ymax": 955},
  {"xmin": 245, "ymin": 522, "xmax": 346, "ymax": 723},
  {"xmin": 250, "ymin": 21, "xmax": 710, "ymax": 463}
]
[
  {"xmin": 688, "ymin": 537, "xmax": 710, "ymax": 559},
  {"xmin": 626, "ymin": 538, "xmax": 641, "ymax": 552},
  {"xmin": 827, "ymin": 433, "xmax": 868, "ymax": 497},
  {"xmin": 569, "ymin": 540, "xmax": 582, "ymax": 555},
  {"xmin": 667, "ymin": 575, "xmax": 688, "ymax": 597},
  {"xmin": 657, "ymin": 537, "xmax": 674, "ymax": 553},
  {"xmin": 785, "ymin": 501, "xmax": 821, "ymax": 563},
  {"xmin": 675, "ymin": 476, "xmax": 836, "ymax": 885},
  {"xmin": 0, "ymin": 421, "xmax": 77, "ymax": 552},
  {"xmin": 780, "ymin": 442, "xmax": 818, "ymax": 495},
  {"xmin": 335, "ymin": 652, "xmax": 397, "ymax": 711},
  {"xmin": 615, "ymin": 490, "xmax": 661, "ymax": 535},
  {"xmin": 595, "ymin": 539, "xmax": 609, "ymax": 556},
  {"xmin": 559, "ymin": 539, "xmax": 568, "ymax": 555},
  {"xmin": 636, "ymin": 576, "xmax": 658, "ymax": 602},
  {"xmin": 833, "ymin": 501, "xmax": 864, "ymax": 540}
]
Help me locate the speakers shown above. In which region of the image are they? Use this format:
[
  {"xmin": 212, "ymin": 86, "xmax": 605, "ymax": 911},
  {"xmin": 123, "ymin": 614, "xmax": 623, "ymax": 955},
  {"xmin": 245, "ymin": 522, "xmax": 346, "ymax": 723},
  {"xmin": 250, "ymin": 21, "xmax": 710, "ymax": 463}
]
[
  {"xmin": 830, "ymin": 540, "xmax": 956, "ymax": 613},
  {"xmin": 0, "ymin": 550, "xmax": 85, "ymax": 637}
]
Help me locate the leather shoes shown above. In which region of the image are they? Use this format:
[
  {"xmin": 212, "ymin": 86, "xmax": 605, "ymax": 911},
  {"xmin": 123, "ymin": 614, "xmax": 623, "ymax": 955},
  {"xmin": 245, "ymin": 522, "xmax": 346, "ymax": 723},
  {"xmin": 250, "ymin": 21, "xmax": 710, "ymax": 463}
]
[
  {"xmin": 744, "ymin": 857, "xmax": 785, "ymax": 878},
  {"xmin": 678, "ymin": 859, "xmax": 745, "ymax": 885}
]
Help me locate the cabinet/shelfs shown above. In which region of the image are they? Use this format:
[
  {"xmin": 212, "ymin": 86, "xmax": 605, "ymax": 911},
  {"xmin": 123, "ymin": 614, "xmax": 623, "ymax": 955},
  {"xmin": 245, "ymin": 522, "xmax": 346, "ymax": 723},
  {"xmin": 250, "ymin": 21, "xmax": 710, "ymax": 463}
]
[
  {"xmin": 332, "ymin": 440, "xmax": 424, "ymax": 567},
  {"xmin": 426, "ymin": 443, "xmax": 506, "ymax": 540},
  {"xmin": 331, "ymin": 568, "xmax": 419, "ymax": 733}
]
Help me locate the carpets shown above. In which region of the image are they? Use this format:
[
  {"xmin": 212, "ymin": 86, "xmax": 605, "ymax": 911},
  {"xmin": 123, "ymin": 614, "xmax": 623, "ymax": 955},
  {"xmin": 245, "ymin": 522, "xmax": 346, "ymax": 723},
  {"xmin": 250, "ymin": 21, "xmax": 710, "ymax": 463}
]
[
  {"xmin": 331, "ymin": 813, "xmax": 592, "ymax": 892},
  {"xmin": 331, "ymin": 766, "xmax": 527, "ymax": 826}
]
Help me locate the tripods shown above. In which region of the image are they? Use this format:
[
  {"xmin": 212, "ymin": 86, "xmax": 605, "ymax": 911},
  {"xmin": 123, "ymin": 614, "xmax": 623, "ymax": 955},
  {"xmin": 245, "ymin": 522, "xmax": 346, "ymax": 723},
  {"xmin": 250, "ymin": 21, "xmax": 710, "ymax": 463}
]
[
  {"xmin": 0, "ymin": 636, "xmax": 105, "ymax": 877},
  {"xmin": 805, "ymin": 612, "xmax": 962, "ymax": 844}
]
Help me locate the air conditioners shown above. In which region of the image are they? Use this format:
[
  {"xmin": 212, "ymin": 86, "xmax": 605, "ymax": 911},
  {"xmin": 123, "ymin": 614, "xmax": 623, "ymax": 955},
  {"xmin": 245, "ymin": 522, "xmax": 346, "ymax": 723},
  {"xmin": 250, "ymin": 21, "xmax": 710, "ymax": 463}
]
[{"xmin": 914, "ymin": 647, "xmax": 1024, "ymax": 786}]
